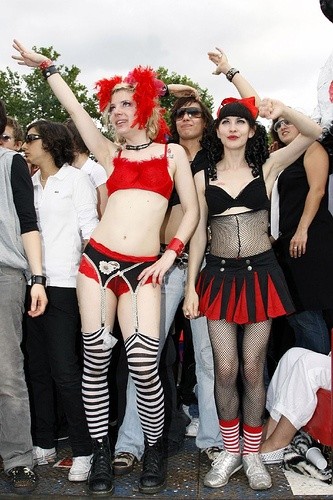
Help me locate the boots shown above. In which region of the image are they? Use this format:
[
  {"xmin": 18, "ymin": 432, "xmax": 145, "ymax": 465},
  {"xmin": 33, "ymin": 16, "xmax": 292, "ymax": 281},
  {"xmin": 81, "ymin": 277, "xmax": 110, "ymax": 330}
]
[
  {"xmin": 88, "ymin": 435, "xmax": 113, "ymax": 497},
  {"xmin": 138, "ymin": 437, "xmax": 167, "ymax": 494}
]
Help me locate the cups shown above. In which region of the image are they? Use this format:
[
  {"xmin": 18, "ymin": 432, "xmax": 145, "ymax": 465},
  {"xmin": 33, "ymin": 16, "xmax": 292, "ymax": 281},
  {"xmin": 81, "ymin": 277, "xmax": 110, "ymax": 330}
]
[{"xmin": 305, "ymin": 447, "xmax": 327, "ymax": 470}]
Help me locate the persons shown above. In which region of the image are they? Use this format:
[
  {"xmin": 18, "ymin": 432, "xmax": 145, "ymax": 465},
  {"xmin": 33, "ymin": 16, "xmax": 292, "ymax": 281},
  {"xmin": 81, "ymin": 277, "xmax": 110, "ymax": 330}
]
[{"xmin": 0, "ymin": 0, "xmax": 333, "ymax": 498}]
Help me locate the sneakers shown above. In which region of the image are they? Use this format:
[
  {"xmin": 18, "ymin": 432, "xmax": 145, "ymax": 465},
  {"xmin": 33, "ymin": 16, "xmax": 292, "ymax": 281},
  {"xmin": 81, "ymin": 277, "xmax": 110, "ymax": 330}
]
[
  {"xmin": 68, "ymin": 453, "xmax": 95, "ymax": 481},
  {"xmin": 204, "ymin": 450, "xmax": 243, "ymax": 488},
  {"xmin": 32, "ymin": 446, "xmax": 56, "ymax": 465},
  {"xmin": 242, "ymin": 453, "xmax": 272, "ymax": 490},
  {"xmin": 112, "ymin": 450, "xmax": 137, "ymax": 475},
  {"xmin": 205, "ymin": 447, "xmax": 221, "ymax": 461}
]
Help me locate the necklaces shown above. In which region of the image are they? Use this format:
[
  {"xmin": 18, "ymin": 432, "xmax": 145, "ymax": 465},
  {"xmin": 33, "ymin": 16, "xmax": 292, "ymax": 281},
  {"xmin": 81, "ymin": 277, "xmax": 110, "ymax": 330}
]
[
  {"xmin": 124, "ymin": 139, "xmax": 153, "ymax": 150},
  {"xmin": 41, "ymin": 175, "xmax": 46, "ymax": 182}
]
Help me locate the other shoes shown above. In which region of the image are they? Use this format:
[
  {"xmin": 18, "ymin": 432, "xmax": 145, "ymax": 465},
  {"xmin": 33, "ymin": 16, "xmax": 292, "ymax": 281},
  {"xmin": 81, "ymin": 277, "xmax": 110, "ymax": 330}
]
[
  {"xmin": 8, "ymin": 466, "xmax": 37, "ymax": 492},
  {"xmin": 167, "ymin": 439, "xmax": 180, "ymax": 458},
  {"xmin": 185, "ymin": 418, "xmax": 199, "ymax": 437},
  {"xmin": 259, "ymin": 446, "xmax": 288, "ymax": 464},
  {"xmin": 53, "ymin": 425, "xmax": 71, "ymax": 441}
]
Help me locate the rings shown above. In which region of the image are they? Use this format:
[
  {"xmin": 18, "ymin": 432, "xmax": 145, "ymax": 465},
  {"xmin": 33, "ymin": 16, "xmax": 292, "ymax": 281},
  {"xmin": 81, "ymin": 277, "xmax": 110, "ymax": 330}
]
[
  {"xmin": 185, "ymin": 312, "xmax": 189, "ymax": 315},
  {"xmin": 293, "ymin": 247, "xmax": 297, "ymax": 249}
]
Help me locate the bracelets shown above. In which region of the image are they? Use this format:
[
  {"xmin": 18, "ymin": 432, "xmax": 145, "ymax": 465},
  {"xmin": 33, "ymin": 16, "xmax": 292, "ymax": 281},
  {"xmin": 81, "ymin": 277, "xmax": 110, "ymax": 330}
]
[
  {"xmin": 226, "ymin": 67, "xmax": 240, "ymax": 82},
  {"xmin": 31, "ymin": 274, "xmax": 47, "ymax": 287},
  {"xmin": 160, "ymin": 86, "xmax": 170, "ymax": 97},
  {"xmin": 39, "ymin": 59, "xmax": 53, "ymax": 70},
  {"xmin": 167, "ymin": 237, "xmax": 186, "ymax": 257},
  {"xmin": 43, "ymin": 64, "xmax": 59, "ymax": 80}
]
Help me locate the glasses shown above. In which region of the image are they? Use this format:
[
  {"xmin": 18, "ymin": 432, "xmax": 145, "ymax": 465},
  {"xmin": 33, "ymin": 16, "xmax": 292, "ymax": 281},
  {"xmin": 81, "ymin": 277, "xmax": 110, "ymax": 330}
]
[
  {"xmin": 274, "ymin": 119, "xmax": 292, "ymax": 132},
  {"xmin": 0, "ymin": 133, "xmax": 15, "ymax": 142},
  {"xmin": 172, "ymin": 107, "xmax": 203, "ymax": 121},
  {"xmin": 25, "ymin": 134, "xmax": 43, "ymax": 144}
]
